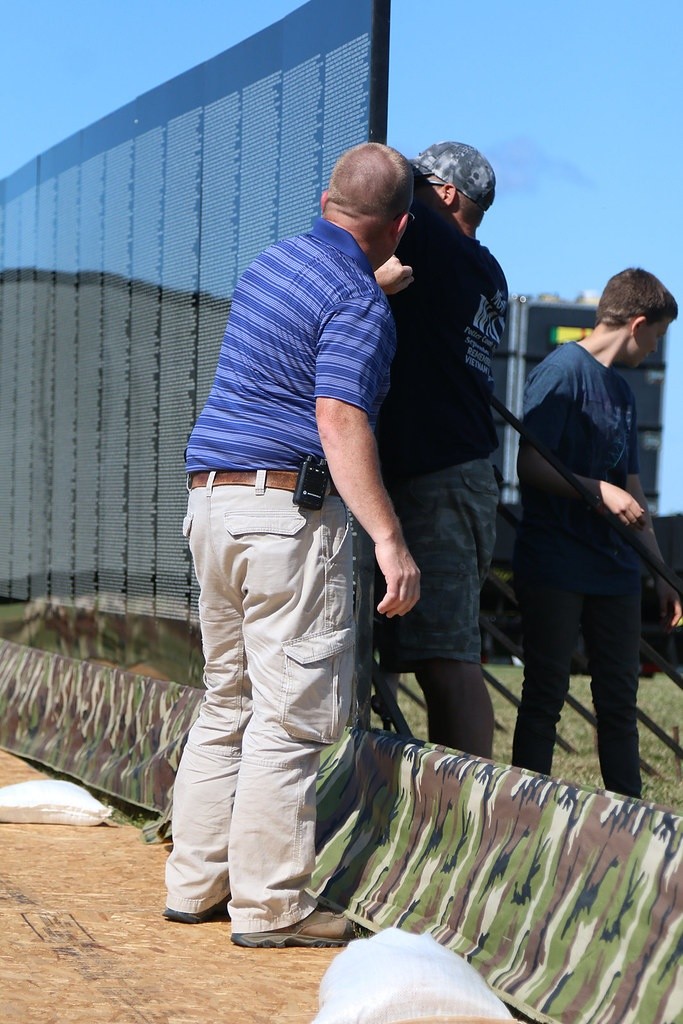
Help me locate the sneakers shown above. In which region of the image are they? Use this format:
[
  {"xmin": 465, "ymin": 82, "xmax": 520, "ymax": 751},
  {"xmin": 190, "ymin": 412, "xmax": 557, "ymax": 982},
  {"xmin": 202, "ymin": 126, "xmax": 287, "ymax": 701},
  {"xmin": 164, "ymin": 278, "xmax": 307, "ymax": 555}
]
[
  {"xmin": 231, "ymin": 910, "xmax": 352, "ymax": 950},
  {"xmin": 162, "ymin": 891, "xmax": 233, "ymax": 927}
]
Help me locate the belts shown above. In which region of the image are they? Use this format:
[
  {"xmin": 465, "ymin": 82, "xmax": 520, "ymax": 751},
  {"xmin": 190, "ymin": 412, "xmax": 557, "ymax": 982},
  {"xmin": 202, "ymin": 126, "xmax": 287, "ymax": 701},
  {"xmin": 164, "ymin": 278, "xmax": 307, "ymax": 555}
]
[{"xmin": 185, "ymin": 466, "xmax": 340, "ymax": 496}]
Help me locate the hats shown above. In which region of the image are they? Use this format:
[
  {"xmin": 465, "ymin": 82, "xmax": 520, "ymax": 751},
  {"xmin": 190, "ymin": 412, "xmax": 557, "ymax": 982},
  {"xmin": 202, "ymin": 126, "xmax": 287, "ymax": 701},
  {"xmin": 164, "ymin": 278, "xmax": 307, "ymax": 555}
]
[{"xmin": 402, "ymin": 141, "xmax": 494, "ymax": 210}]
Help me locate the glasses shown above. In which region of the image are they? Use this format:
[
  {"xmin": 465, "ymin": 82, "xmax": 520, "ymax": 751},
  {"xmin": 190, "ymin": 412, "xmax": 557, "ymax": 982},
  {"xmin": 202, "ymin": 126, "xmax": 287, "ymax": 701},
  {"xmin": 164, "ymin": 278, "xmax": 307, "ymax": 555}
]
[
  {"xmin": 396, "ymin": 210, "xmax": 416, "ymax": 224},
  {"xmin": 412, "ymin": 177, "xmax": 469, "ymax": 197}
]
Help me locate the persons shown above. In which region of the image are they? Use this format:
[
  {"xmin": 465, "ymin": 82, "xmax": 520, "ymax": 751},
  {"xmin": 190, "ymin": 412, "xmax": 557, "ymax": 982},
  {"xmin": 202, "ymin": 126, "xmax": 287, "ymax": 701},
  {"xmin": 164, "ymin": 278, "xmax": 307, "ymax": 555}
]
[
  {"xmin": 368, "ymin": 142, "xmax": 510, "ymax": 760},
  {"xmin": 163, "ymin": 142, "xmax": 424, "ymax": 944},
  {"xmin": 513, "ymin": 266, "xmax": 682, "ymax": 799}
]
